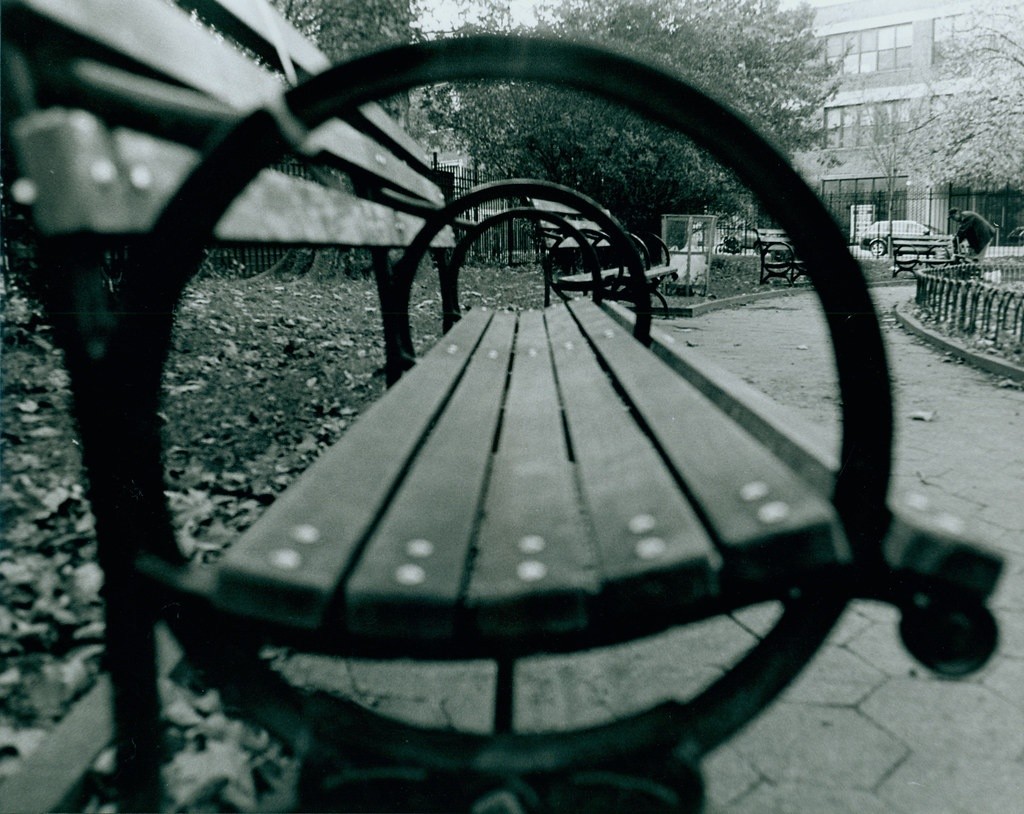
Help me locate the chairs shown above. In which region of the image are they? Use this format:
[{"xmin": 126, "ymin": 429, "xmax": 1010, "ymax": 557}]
[{"xmin": 0, "ymin": 0, "xmax": 1002, "ymax": 814}]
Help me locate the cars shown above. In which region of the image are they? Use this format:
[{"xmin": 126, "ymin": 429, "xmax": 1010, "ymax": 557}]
[
  {"xmin": 860, "ymin": 221, "xmax": 946, "ymax": 256},
  {"xmin": 1007, "ymin": 227, "xmax": 1024, "ymax": 246}
]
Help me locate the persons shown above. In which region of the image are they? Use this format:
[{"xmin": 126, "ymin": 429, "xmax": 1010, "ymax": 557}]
[{"xmin": 947, "ymin": 207, "xmax": 997, "ymax": 281}]
